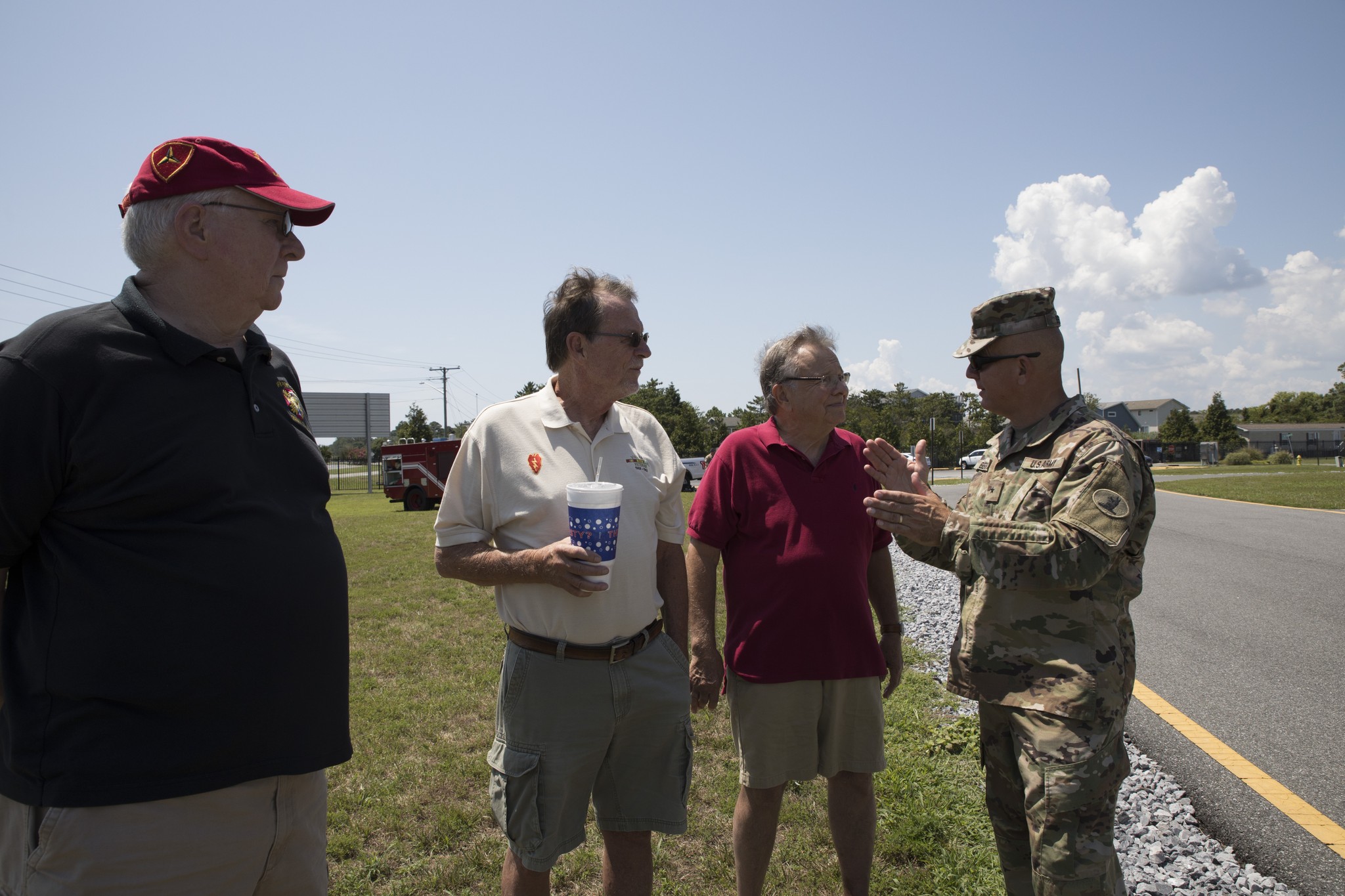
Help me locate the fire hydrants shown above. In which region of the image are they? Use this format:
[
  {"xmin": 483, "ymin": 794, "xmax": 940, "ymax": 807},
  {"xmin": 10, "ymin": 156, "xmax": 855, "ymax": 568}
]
[{"xmin": 1296, "ymin": 455, "xmax": 1303, "ymax": 466}]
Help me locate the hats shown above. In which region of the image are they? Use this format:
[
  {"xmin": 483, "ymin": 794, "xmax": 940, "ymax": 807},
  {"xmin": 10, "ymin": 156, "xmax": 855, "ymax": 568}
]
[
  {"xmin": 952, "ymin": 286, "xmax": 1061, "ymax": 358},
  {"xmin": 119, "ymin": 136, "xmax": 335, "ymax": 228}
]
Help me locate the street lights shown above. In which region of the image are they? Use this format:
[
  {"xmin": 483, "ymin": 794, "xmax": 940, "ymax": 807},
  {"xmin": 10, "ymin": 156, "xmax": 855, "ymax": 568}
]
[{"xmin": 419, "ymin": 381, "xmax": 448, "ymax": 438}]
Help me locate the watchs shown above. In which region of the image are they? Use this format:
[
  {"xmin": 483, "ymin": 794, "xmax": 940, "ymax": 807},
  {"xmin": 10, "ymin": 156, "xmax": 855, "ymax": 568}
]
[{"xmin": 880, "ymin": 621, "xmax": 905, "ymax": 637}]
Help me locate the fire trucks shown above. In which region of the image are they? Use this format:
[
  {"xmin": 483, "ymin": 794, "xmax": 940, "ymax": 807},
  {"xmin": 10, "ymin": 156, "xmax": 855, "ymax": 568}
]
[{"xmin": 381, "ymin": 431, "xmax": 463, "ymax": 512}]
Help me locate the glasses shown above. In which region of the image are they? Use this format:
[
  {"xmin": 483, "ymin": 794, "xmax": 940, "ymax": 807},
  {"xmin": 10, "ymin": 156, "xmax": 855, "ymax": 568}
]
[
  {"xmin": 968, "ymin": 352, "xmax": 1041, "ymax": 370},
  {"xmin": 589, "ymin": 331, "xmax": 651, "ymax": 349},
  {"xmin": 777, "ymin": 372, "xmax": 850, "ymax": 391},
  {"xmin": 201, "ymin": 201, "xmax": 295, "ymax": 236}
]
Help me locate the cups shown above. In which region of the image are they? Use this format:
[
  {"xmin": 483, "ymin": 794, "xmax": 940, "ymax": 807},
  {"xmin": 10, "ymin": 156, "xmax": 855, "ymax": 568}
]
[{"xmin": 566, "ymin": 482, "xmax": 625, "ymax": 592}]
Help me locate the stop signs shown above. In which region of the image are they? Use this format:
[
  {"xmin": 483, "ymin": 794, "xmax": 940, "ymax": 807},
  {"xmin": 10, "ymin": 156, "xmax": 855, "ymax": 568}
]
[{"xmin": 1168, "ymin": 445, "xmax": 1175, "ymax": 453}]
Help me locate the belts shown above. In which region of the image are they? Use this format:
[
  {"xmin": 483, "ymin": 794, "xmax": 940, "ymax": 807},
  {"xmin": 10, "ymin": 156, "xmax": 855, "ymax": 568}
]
[{"xmin": 506, "ymin": 617, "xmax": 666, "ymax": 664}]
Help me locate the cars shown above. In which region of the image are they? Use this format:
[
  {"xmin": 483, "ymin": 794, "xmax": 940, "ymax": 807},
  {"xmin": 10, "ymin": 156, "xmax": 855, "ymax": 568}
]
[
  {"xmin": 900, "ymin": 452, "xmax": 931, "ymax": 469},
  {"xmin": 1144, "ymin": 455, "xmax": 1153, "ymax": 467}
]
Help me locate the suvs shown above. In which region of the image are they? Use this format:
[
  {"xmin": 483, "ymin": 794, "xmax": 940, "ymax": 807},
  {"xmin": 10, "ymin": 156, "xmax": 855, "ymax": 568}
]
[{"xmin": 959, "ymin": 449, "xmax": 988, "ymax": 470}]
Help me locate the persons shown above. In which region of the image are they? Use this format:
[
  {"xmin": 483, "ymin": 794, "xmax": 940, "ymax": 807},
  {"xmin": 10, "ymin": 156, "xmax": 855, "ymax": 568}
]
[
  {"xmin": 686, "ymin": 323, "xmax": 903, "ymax": 896},
  {"xmin": 435, "ymin": 266, "xmax": 694, "ymax": 896},
  {"xmin": 0, "ymin": 137, "xmax": 353, "ymax": 895},
  {"xmin": 863, "ymin": 287, "xmax": 1156, "ymax": 896}
]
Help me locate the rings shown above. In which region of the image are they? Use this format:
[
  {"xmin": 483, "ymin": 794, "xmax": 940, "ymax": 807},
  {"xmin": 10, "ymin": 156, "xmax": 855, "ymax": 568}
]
[{"xmin": 899, "ymin": 513, "xmax": 903, "ymax": 522}]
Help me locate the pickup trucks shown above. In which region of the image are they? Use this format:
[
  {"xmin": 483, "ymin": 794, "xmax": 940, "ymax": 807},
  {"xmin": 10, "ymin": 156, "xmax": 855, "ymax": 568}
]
[{"xmin": 680, "ymin": 457, "xmax": 706, "ymax": 488}]
